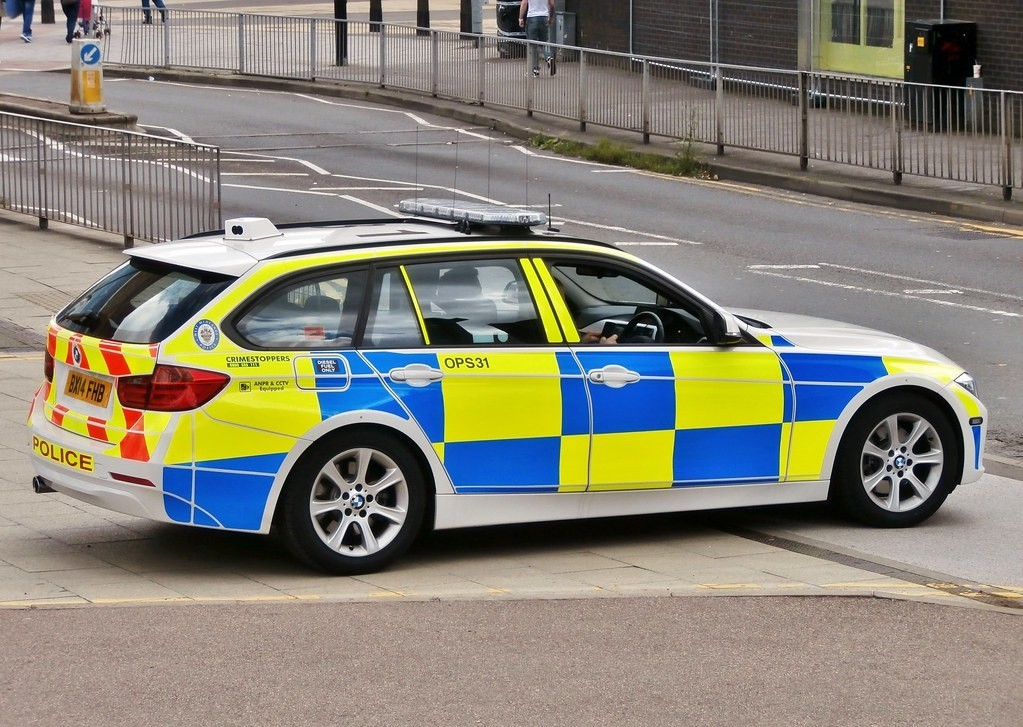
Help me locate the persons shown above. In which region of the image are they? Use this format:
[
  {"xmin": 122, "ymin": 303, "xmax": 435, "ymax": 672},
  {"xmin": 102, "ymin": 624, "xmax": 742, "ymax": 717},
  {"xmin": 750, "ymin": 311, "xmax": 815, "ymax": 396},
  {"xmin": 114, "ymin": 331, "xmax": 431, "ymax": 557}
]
[
  {"xmin": 142, "ymin": 0, "xmax": 166, "ymax": 24},
  {"xmin": 438, "ymin": 266, "xmax": 482, "ymax": 299},
  {"xmin": 61, "ymin": 0, "xmax": 92, "ymax": 43},
  {"xmin": 18, "ymin": 0, "xmax": 35, "ymax": 42},
  {"xmin": 554, "ymin": 277, "xmax": 655, "ymax": 344},
  {"xmin": 519, "ymin": 0, "xmax": 556, "ymax": 77}
]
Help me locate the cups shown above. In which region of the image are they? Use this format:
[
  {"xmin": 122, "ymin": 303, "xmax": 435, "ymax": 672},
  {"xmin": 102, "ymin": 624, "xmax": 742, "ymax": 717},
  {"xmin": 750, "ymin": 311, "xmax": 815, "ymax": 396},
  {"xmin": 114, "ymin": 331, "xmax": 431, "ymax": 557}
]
[{"xmin": 973, "ymin": 64, "xmax": 982, "ymax": 78}]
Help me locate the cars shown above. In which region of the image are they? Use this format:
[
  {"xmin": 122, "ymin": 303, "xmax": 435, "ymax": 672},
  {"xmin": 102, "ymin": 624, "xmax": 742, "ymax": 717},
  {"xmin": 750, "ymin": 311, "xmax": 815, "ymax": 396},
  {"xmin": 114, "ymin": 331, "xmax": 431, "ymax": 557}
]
[{"xmin": 24, "ymin": 197, "xmax": 990, "ymax": 580}]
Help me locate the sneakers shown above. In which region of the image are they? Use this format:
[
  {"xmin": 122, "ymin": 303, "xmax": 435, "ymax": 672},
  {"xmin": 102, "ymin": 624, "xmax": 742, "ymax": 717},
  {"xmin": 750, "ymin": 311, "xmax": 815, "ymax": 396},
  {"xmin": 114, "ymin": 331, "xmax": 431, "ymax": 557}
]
[{"xmin": 20, "ymin": 32, "xmax": 33, "ymax": 43}]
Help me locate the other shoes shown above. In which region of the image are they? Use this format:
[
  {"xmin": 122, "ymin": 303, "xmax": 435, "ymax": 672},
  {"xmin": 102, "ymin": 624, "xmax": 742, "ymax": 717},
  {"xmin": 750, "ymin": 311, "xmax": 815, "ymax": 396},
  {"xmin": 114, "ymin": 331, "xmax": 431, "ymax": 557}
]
[
  {"xmin": 142, "ymin": 16, "xmax": 153, "ymax": 25},
  {"xmin": 159, "ymin": 9, "xmax": 168, "ymax": 23},
  {"xmin": 66, "ymin": 37, "xmax": 73, "ymax": 43}
]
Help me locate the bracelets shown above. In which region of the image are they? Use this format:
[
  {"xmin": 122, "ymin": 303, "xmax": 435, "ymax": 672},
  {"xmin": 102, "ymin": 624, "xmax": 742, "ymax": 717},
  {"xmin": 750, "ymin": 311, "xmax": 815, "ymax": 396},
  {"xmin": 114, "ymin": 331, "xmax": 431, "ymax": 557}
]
[{"xmin": 519, "ymin": 19, "xmax": 523, "ymax": 21}]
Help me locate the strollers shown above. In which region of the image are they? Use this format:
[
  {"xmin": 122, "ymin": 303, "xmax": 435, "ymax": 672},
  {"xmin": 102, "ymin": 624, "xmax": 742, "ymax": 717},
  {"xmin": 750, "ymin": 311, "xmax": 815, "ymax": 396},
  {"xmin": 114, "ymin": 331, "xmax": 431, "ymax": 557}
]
[{"xmin": 73, "ymin": 5, "xmax": 111, "ymax": 39}]
